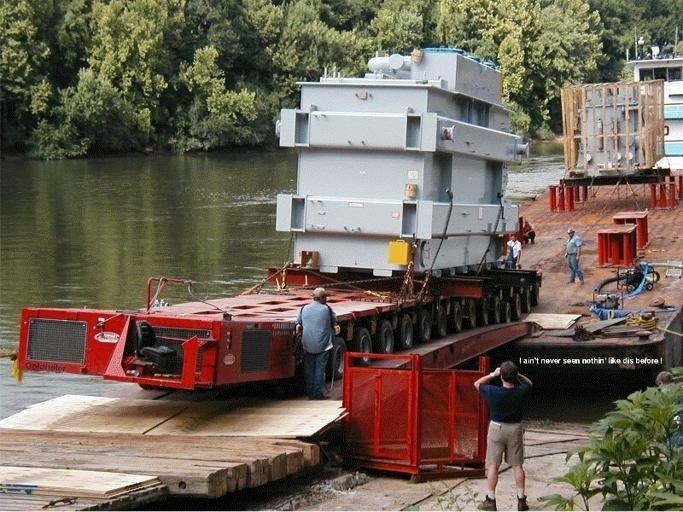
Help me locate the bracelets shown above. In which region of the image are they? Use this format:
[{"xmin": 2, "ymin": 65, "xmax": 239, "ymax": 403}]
[{"xmin": 489, "ymin": 372, "xmax": 495, "ymax": 378}]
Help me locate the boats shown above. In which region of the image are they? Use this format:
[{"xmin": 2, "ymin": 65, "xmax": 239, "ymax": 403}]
[{"xmin": 506, "ymin": 21, "xmax": 683, "ymax": 386}]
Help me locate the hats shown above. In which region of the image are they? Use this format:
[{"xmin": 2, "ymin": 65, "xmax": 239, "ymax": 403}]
[{"xmin": 310, "ymin": 287, "xmax": 329, "ymax": 297}]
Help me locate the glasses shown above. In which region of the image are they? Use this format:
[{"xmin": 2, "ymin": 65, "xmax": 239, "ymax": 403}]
[{"xmin": 568, "ymin": 232, "xmax": 571, "ymax": 234}]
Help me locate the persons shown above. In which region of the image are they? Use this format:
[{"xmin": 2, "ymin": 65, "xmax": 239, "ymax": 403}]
[
  {"xmin": 564, "ymin": 228, "xmax": 585, "ymax": 286},
  {"xmin": 506, "ymin": 233, "xmax": 522, "ymax": 269},
  {"xmin": 522, "ymin": 219, "xmax": 536, "ymax": 244},
  {"xmin": 474, "ymin": 360, "xmax": 534, "ymax": 511},
  {"xmin": 655, "ymin": 370, "xmax": 683, "ymax": 448},
  {"xmin": 297, "ymin": 287, "xmax": 337, "ymax": 399}
]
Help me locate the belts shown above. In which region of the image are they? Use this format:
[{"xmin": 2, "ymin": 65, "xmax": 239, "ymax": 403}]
[{"xmin": 568, "ymin": 253, "xmax": 576, "ymax": 255}]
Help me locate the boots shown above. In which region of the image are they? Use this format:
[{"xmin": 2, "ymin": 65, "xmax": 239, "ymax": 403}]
[
  {"xmin": 517, "ymin": 494, "xmax": 529, "ymax": 510},
  {"xmin": 478, "ymin": 496, "xmax": 496, "ymax": 511}
]
